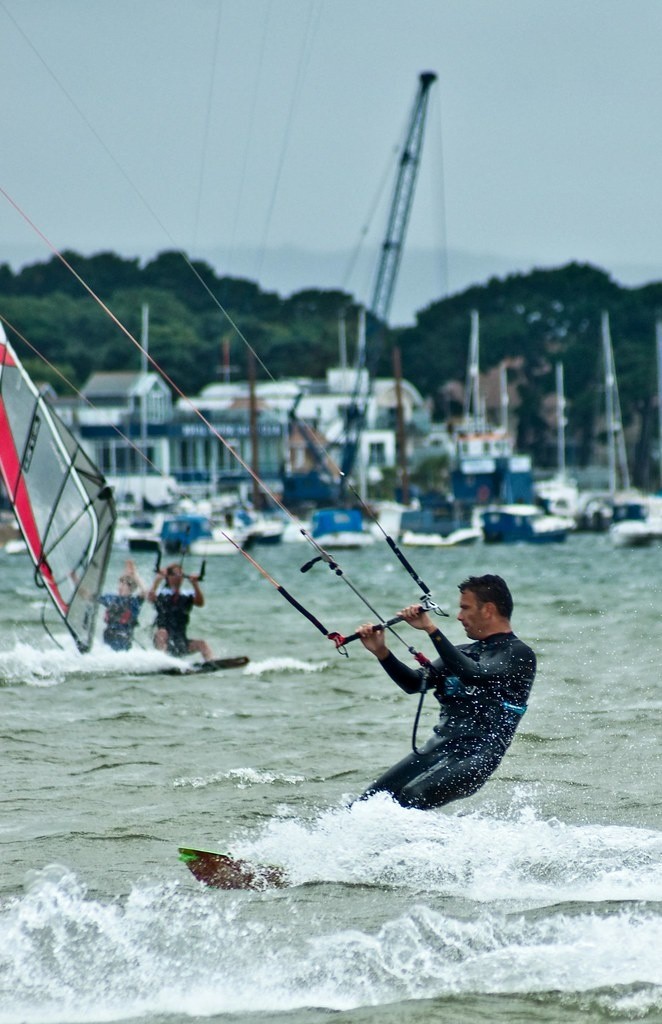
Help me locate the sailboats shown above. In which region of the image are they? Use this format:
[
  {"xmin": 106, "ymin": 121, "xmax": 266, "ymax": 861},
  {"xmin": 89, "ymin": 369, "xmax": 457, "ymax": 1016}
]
[{"xmin": 1, "ymin": 324, "xmax": 253, "ymax": 685}]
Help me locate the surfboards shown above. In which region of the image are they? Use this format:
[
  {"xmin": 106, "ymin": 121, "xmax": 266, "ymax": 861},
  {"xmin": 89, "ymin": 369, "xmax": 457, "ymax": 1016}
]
[
  {"xmin": 176, "ymin": 845, "xmax": 289, "ymax": 891},
  {"xmin": 123, "ymin": 656, "xmax": 250, "ymax": 677}
]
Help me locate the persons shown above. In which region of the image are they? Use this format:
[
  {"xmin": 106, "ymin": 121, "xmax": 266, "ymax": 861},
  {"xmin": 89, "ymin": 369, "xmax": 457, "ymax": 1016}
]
[
  {"xmin": 147, "ymin": 563, "xmax": 212, "ymax": 661},
  {"xmin": 69, "ymin": 559, "xmax": 146, "ymax": 653},
  {"xmin": 345, "ymin": 575, "xmax": 536, "ymax": 812}
]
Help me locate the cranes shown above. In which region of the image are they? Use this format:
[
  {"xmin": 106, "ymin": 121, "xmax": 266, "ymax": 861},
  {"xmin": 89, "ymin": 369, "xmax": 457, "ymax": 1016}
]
[{"xmin": 283, "ymin": 69, "xmax": 440, "ymax": 506}]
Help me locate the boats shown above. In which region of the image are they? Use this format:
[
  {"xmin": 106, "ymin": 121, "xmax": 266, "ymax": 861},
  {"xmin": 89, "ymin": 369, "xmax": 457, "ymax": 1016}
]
[{"xmin": 116, "ymin": 505, "xmax": 661, "ymax": 558}]
